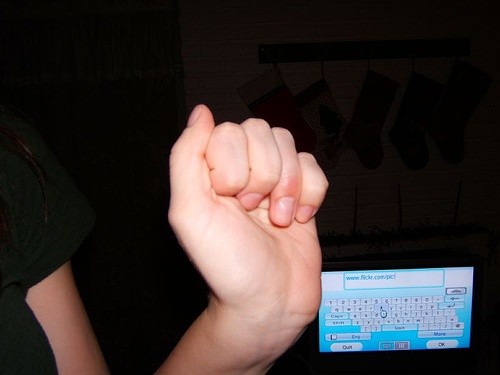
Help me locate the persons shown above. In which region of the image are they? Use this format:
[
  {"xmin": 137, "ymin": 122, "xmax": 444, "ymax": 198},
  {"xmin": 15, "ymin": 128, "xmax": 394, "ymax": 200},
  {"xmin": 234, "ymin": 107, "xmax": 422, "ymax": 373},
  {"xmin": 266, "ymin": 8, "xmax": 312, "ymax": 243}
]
[{"xmin": 0, "ymin": 104, "xmax": 330, "ymax": 375}]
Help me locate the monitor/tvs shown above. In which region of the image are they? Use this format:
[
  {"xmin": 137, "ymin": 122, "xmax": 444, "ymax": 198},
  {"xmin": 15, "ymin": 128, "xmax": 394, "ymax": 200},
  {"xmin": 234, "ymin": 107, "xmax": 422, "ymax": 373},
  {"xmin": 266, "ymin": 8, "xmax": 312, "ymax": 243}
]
[{"xmin": 313, "ymin": 251, "xmax": 491, "ymax": 375}]
